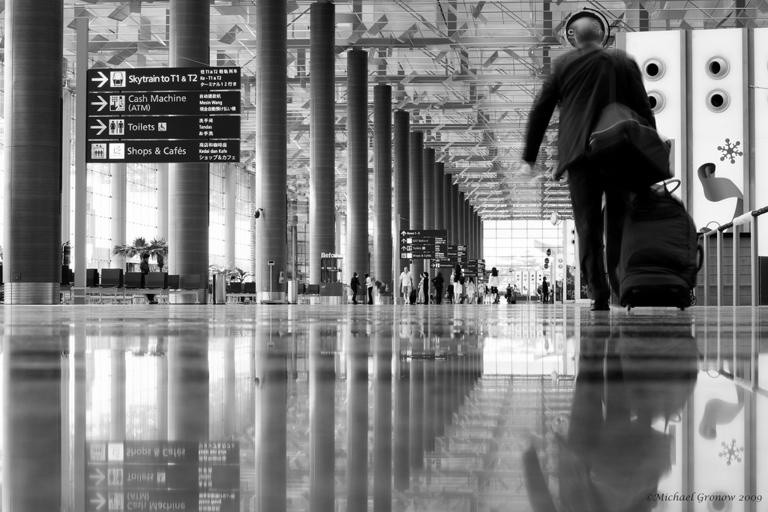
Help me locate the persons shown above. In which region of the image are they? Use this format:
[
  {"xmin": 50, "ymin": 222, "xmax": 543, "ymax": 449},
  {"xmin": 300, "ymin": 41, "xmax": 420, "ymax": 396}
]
[
  {"xmin": 397, "ymin": 266, "xmax": 413, "ymax": 304},
  {"xmin": 521, "ymin": 322, "xmax": 660, "ymax": 510},
  {"xmin": 140, "ymin": 254, "xmax": 151, "ymax": 273},
  {"xmin": 416, "ymin": 272, "xmax": 425, "ymax": 304},
  {"xmin": 431, "ymin": 272, "xmax": 444, "ymax": 305},
  {"xmin": 541, "ymin": 276, "xmax": 548, "ymax": 303},
  {"xmin": 362, "ymin": 273, "xmax": 373, "ymax": 304},
  {"xmin": 519, "ymin": 18, "xmax": 657, "ymax": 311},
  {"xmin": 350, "ymin": 272, "xmax": 363, "ymax": 304},
  {"xmin": 422, "ymin": 272, "xmax": 429, "ymax": 305},
  {"xmin": 446, "ymin": 264, "xmax": 515, "ymax": 305}
]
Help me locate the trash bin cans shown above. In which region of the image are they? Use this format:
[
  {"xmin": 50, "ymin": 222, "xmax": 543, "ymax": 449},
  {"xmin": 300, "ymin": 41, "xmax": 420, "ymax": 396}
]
[
  {"xmin": 288, "ymin": 280, "xmax": 298, "ymax": 304},
  {"xmin": 212, "ymin": 273, "xmax": 226, "ymax": 305}
]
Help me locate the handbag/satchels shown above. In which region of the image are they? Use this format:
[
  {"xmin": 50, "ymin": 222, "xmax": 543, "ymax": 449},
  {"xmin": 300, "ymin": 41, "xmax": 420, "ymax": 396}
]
[{"xmin": 589, "ymin": 103, "xmax": 674, "ymax": 187}]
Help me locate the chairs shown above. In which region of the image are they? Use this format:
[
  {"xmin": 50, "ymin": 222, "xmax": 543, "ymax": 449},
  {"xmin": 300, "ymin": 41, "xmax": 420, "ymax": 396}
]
[{"xmin": 61, "ymin": 264, "xmax": 320, "ymax": 304}]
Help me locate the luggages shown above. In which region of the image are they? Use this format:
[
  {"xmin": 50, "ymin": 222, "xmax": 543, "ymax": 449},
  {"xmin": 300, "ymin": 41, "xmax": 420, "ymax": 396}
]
[{"xmin": 617, "ymin": 186, "xmax": 702, "ymax": 310}]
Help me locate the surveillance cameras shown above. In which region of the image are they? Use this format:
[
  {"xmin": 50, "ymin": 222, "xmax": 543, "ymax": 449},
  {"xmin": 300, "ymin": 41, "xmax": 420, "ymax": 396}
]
[
  {"xmin": 410, "ymin": 260, "xmax": 412, "ymax": 264},
  {"xmin": 254, "ymin": 210, "xmax": 260, "ymax": 218}
]
